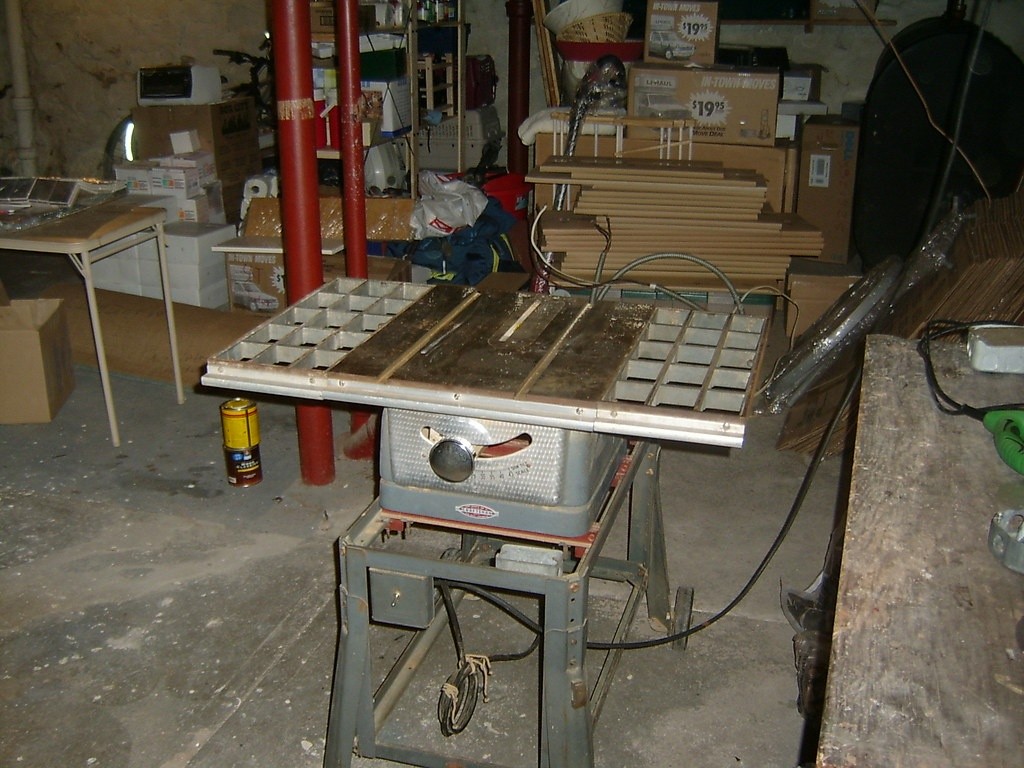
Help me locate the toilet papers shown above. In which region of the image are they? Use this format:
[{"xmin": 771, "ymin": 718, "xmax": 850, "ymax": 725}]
[{"xmin": 239, "ymin": 172, "xmax": 278, "ymax": 228}]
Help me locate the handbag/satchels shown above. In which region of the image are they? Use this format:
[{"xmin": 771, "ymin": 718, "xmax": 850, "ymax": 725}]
[{"xmin": 408, "ymin": 170, "xmax": 489, "ymax": 239}]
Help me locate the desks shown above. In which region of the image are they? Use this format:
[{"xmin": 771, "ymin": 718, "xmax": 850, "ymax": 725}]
[
  {"xmin": 0, "ymin": 208, "xmax": 186, "ymax": 446},
  {"xmin": 814, "ymin": 334, "xmax": 1024, "ymax": 768}
]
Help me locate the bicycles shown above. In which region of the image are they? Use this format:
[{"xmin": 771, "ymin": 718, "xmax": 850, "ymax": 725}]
[{"xmin": 104, "ymin": 39, "xmax": 275, "ymax": 192}]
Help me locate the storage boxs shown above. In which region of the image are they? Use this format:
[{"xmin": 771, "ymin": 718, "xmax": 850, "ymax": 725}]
[
  {"xmin": 86, "ymin": 0, "xmax": 530, "ymax": 315},
  {"xmin": 0, "ymin": 296, "xmax": 76, "ymax": 425},
  {"xmin": 535, "ymin": 0, "xmax": 860, "ymax": 264}
]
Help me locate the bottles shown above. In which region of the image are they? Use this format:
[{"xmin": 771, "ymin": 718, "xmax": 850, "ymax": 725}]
[{"xmin": 219, "ymin": 397, "xmax": 262, "ymax": 489}]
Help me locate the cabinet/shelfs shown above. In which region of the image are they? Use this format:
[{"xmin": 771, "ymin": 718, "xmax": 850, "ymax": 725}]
[{"xmin": 212, "ymin": 0, "xmax": 467, "ymax": 255}]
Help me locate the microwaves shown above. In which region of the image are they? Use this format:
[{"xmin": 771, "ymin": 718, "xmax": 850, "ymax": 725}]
[{"xmin": 135, "ymin": 62, "xmax": 223, "ymax": 107}]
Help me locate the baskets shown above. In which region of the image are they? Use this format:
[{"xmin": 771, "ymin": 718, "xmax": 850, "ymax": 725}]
[{"xmin": 556, "ymin": 12, "xmax": 634, "ymax": 42}]
